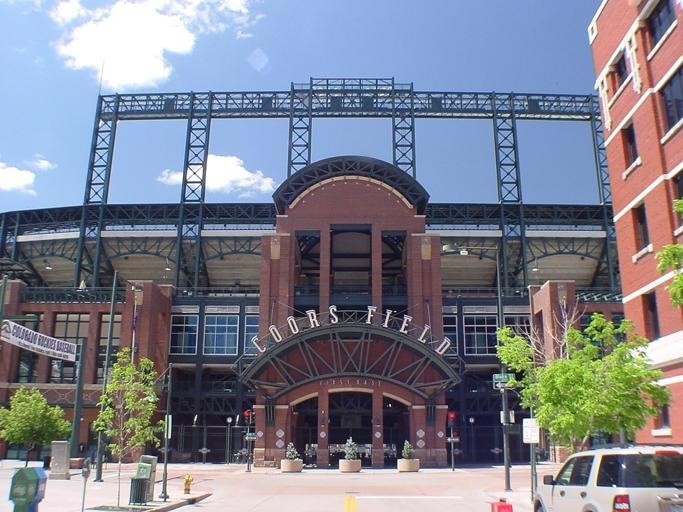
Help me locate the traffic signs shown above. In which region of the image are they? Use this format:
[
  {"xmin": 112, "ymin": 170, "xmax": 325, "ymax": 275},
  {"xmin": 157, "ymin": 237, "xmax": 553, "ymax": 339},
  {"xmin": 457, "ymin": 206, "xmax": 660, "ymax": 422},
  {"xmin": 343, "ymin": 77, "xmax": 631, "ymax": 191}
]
[{"xmin": 493, "ymin": 374, "xmax": 516, "ymax": 383}]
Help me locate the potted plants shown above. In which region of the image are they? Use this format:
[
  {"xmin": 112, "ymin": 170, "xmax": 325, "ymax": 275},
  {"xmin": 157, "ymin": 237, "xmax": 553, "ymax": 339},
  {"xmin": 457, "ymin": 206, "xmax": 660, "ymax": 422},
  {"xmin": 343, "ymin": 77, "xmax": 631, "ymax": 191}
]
[
  {"xmin": 397, "ymin": 440, "xmax": 420, "ymax": 472},
  {"xmin": 339, "ymin": 437, "xmax": 361, "ymax": 473},
  {"xmin": 281, "ymin": 442, "xmax": 302, "ymax": 473}
]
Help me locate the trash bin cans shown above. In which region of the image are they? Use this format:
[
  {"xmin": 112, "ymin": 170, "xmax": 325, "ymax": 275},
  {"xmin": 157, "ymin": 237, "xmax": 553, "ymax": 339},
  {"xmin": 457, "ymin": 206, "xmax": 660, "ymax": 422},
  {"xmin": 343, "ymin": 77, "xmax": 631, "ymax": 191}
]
[
  {"xmin": 44, "ymin": 456, "xmax": 51, "ymax": 468},
  {"xmin": 128, "ymin": 478, "xmax": 148, "ymax": 505}
]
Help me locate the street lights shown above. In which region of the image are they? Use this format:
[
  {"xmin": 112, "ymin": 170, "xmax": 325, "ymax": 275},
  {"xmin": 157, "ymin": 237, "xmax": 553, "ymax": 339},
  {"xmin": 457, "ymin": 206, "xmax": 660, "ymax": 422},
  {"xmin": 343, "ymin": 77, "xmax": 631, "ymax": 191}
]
[
  {"xmin": 443, "ymin": 245, "xmax": 511, "ymax": 489},
  {"xmin": 93, "ymin": 271, "xmax": 144, "ymax": 482}
]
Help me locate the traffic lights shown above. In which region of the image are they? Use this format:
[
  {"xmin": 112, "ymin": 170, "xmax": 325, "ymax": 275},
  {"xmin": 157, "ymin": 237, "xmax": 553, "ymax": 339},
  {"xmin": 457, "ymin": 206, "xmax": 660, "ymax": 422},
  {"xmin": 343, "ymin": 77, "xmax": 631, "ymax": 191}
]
[
  {"xmin": 447, "ymin": 410, "xmax": 457, "ymax": 429},
  {"xmin": 243, "ymin": 410, "xmax": 256, "ymax": 426}
]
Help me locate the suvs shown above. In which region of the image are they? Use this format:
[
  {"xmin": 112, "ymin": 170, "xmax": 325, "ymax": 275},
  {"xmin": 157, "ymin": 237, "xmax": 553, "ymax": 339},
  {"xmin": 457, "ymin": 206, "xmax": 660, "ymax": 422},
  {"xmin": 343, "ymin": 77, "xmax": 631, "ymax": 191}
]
[{"xmin": 533, "ymin": 443, "xmax": 683, "ymax": 512}]
